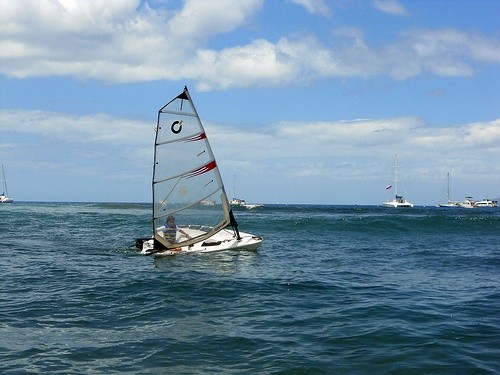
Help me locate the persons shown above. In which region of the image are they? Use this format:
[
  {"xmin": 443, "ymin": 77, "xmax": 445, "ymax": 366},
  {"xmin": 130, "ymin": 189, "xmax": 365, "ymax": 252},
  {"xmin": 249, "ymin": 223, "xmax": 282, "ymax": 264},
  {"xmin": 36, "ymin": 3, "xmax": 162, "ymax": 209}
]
[{"xmin": 154, "ymin": 216, "xmax": 193, "ymax": 246}]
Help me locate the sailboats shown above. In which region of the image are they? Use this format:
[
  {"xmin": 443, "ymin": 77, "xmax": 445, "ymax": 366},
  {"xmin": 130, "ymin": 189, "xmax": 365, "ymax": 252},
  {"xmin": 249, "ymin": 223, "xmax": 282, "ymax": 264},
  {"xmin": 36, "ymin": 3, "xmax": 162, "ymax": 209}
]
[
  {"xmin": 383, "ymin": 153, "xmax": 413, "ymax": 208},
  {"xmin": 133, "ymin": 86, "xmax": 264, "ymax": 260}
]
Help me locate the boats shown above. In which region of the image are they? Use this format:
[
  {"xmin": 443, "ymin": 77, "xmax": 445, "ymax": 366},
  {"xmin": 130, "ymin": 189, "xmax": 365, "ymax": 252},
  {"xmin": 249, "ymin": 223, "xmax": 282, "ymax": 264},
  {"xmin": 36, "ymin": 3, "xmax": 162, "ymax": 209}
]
[
  {"xmin": 439, "ymin": 197, "xmax": 496, "ymax": 208},
  {"xmin": 0, "ymin": 195, "xmax": 13, "ymax": 203},
  {"xmin": 228, "ymin": 199, "xmax": 264, "ymax": 208}
]
